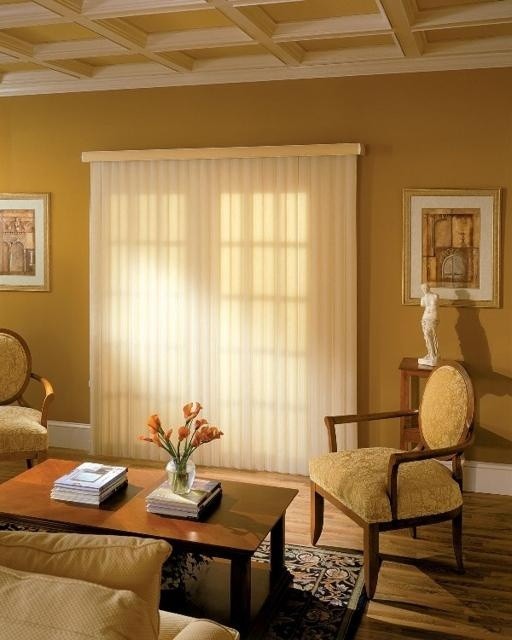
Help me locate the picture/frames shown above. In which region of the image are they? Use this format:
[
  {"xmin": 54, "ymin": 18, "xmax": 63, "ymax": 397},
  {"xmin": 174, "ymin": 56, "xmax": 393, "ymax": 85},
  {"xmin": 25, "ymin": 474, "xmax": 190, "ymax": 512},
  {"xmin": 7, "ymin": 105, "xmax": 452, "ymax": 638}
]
[
  {"xmin": 401, "ymin": 186, "xmax": 504, "ymax": 309},
  {"xmin": 0, "ymin": 191, "xmax": 53, "ymax": 293}
]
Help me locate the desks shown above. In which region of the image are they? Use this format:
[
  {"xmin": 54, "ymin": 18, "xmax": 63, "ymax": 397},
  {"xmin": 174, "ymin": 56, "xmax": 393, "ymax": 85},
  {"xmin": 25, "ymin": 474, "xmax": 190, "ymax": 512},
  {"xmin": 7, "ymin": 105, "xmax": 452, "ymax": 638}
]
[{"xmin": 398, "ymin": 356, "xmax": 465, "ymax": 493}]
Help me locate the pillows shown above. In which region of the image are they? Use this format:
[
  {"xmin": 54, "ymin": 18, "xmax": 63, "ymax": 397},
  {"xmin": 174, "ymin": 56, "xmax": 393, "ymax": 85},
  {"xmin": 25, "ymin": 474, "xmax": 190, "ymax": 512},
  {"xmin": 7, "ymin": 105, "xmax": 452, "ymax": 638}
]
[{"xmin": 0, "ymin": 531, "xmax": 172, "ymax": 640}]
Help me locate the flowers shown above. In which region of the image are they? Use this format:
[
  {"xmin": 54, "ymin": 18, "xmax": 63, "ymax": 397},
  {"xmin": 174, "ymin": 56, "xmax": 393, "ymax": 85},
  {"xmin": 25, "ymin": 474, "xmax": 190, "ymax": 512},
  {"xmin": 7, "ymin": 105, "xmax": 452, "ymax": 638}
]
[{"xmin": 136, "ymin": 401, "xmax": 224, "ymax": 491}]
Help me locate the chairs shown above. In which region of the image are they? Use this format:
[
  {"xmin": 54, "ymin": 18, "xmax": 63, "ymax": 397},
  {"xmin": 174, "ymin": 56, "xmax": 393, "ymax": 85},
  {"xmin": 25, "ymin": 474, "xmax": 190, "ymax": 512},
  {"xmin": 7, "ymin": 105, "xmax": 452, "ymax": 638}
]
[
  {"xmin": 0, "ymin": 328, "xmax": 56, "ymax": 468},
  {"xmin": 309, "ymin": 358, "xmax": 475, "ymax": 599},
  {"xmin": 0, "ymin": 531, "xmax": 240, "ymax": 640}
]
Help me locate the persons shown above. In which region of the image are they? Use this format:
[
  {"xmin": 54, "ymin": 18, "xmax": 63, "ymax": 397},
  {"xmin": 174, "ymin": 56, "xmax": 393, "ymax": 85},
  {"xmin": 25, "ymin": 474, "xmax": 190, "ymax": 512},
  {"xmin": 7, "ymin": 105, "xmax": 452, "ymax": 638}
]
[{"xmin": 418, "ymin": 283, "xmax": 440, "ymax": 361}]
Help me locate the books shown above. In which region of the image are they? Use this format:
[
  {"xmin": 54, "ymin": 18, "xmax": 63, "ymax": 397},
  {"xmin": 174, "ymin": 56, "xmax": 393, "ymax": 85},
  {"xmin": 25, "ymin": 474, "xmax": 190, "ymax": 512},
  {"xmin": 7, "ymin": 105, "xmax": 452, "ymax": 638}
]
[
  {"xmin": 50, "ymin": 461, "xmax": 129, "ymax": 507},
  {"xmin": 147, "ymin": 477, "xmax": 223, "ymax": 521}
]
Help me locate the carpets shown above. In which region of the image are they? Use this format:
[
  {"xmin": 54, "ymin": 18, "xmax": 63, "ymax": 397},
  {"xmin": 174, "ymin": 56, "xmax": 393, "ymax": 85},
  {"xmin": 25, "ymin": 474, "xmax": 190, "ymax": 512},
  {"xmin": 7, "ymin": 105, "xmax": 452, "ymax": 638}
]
[{"xmin": 162, "ymin": 539, "xmax": 366, "ymax": 640}]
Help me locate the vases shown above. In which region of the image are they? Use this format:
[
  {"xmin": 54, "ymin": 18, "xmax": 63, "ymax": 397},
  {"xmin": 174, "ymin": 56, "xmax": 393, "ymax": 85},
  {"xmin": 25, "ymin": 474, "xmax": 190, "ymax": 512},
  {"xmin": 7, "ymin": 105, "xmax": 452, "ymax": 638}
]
[{"xmin": 166, "ymin": 457, "xmax": 196, "ymax": 494}]
